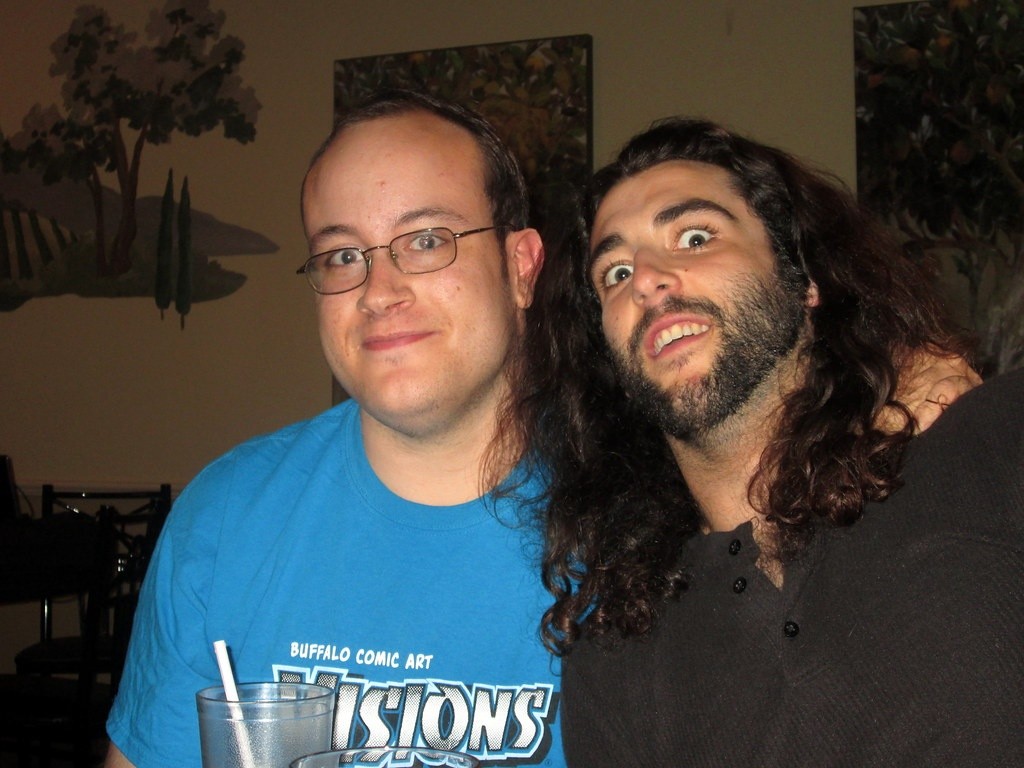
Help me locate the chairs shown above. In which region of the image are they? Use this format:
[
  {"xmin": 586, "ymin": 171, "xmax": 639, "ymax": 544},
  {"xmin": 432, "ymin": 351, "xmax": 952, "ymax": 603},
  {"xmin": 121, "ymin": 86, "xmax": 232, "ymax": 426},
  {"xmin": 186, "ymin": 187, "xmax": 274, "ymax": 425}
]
[{"xmin": 0, "ymin": 482, "xmax": 172, "ymax": 768}]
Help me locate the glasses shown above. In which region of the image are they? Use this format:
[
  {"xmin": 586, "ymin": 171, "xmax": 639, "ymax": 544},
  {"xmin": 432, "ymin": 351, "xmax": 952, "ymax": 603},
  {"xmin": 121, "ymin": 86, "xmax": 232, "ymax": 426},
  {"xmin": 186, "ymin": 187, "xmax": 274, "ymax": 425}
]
[{"xmin": 296, "ymin": 223, "xmax": 496, "ymax": 296}]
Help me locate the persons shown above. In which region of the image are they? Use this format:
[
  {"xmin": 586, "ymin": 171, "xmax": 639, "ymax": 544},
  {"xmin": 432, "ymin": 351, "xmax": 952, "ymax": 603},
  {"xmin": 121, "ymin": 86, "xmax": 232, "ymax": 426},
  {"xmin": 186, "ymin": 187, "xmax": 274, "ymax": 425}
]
[
  {"xmin": 105, "ymin": 89, "xmax": 983, "ymax": 768},
  {"xmin": 560, "ymin": 118, "xmax": 1024, "ymax": 768}
]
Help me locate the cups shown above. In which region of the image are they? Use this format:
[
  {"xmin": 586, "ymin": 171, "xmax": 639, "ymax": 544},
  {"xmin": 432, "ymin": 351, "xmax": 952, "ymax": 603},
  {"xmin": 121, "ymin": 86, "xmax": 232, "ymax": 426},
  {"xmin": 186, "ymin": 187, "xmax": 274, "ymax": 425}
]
[
  {"xmin": 289, "ymin": 746, "xmax": 480, "ymax": 768},
  {"xmin": 195, "ymin": 681, "xmax": 335, "ymax": 768}
]
